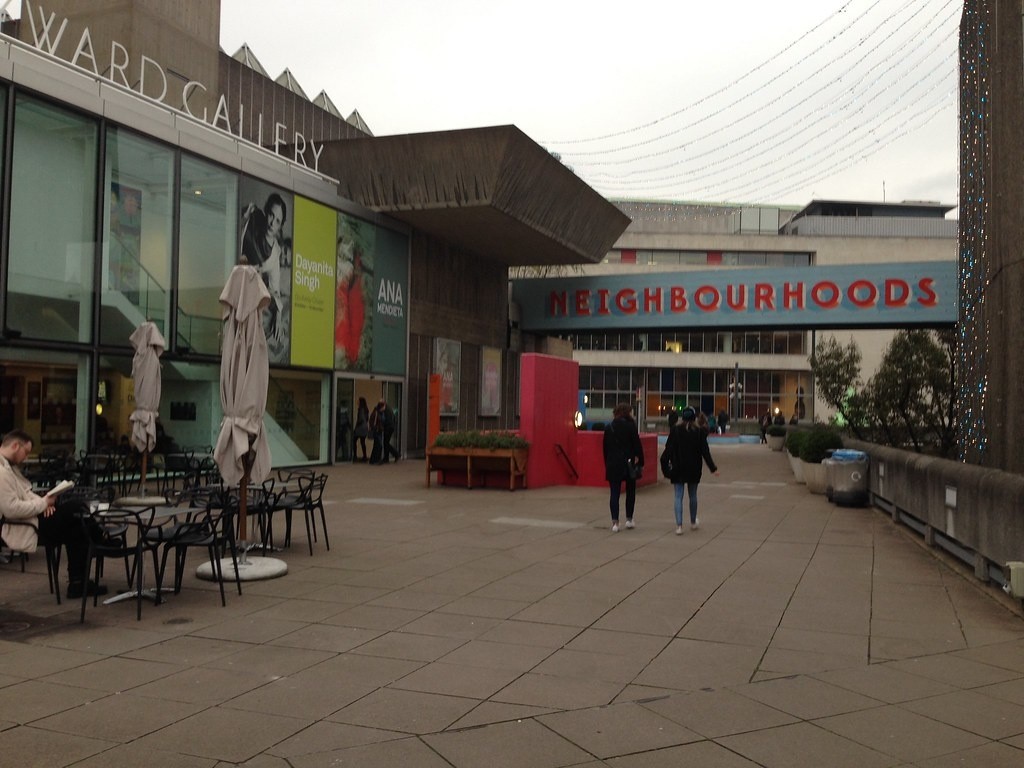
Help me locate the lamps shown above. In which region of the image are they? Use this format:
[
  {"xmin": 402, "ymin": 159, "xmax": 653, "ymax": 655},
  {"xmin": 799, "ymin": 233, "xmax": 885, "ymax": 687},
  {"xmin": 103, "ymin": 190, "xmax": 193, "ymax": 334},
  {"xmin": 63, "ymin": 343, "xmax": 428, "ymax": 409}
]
[{"xmin": 575, "ymin": 411, "xmax": 583, "ymax": 427}]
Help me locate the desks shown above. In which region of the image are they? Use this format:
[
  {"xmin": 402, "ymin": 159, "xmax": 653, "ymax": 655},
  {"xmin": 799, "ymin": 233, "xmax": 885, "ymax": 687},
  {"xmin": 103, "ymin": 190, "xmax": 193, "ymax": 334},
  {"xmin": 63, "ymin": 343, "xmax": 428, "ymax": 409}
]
[
  {"xmin": 79, "ymin": 507, "xmax": 203, "ymax": 606},
  {"xmin": 85, "ymin": 454, "xmax": 126, "ymax": 461},
  {"xmin": 204, "ymin": 482, "xmax": 294, "ymax": 551},
  {"xmin": 167, "ymin": 453, "xmax": 213, "ymax": 496},
  {"xmin": 21, "ymin": 457, "xmax": 57, "ymax": 463}
]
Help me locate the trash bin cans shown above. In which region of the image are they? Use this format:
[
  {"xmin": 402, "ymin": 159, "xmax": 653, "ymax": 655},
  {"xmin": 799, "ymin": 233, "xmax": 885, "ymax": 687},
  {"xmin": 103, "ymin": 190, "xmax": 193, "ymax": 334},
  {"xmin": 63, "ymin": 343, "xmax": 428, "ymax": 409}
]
[{"xmin": 824, "ymin": 448, "xmax": 871, "ymax": 509}]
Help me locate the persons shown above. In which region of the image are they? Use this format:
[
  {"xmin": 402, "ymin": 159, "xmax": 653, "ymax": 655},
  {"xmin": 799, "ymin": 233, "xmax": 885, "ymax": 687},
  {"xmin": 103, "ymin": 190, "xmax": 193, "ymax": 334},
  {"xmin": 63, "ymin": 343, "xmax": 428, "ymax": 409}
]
[
  {"xmin": 336, "ymin": 399, "xmax": 352, "ymax": 461},
  {"xmin": 354, "ymin": 397, "xmax": 369, "ymax": 462},
  {"xmin": 668, "ymin": 406, "xmax": 729, "ymax": 434},
  {"xmin": 0, "ymin": 429, "xmax": 123, "ymax": 598},
  {"xmin": 603, "ymin": 403, "xmax": 645, "ymax": 532},
  {"xmin": 368, "ymin": 399, "xmax": 402, "ymax": 465},
  {"xmin": 661, "ymin": 407, "xmax": 720, "ymax": 535},
  {"xmin": 759, "ymin": 408, "xmax": 798, "ymax": 444},
  {"xmin": 240, "ymin": 193, "xmax": 287, "ymax": 343}
]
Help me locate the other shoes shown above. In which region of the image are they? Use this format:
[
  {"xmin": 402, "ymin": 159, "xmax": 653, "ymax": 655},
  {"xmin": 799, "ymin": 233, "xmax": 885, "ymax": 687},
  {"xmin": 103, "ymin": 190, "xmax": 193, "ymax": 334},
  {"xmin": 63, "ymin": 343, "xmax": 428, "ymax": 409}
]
[
  {"xmin": 353, "ymin": 453, "xmax": 402, "ymax": 465},
  {"xmin": 625, "ymin": 518, "xmax": 634, "ymax": 527},
  {"xmin": 611, "ymin": 521, "xmax": 619, "ymax": 532},
  {"xmin": 692, "ymin": 518, "xmax": 699, "ymax": 529},
  {"xmin": 676, "ymin": 524, "xmax": 682, "ymax": 535},
  {"xmin": 92, "ymin": 537, "xmax": 122, "ymax": 558},
  {"xmin": 65, "ymin": 580, "xmax": 109, "ymax": 599}
]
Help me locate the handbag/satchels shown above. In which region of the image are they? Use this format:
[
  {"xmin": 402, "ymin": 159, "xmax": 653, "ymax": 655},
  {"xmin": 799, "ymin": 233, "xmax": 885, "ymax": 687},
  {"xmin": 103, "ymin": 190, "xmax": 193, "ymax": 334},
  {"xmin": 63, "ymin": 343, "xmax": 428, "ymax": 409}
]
[
  {"xmin": 621, "ymin": 457, "xmax": 640, "ymax": 480},
  {"xmin": 717, "ymin": 426, "xmax": 722, "ymax": 436},
  {"xmin": 762, "ymin": 415, "xmax": 768, "ymax": 428},
  {"xmin": 661, "ymin": 426, "xmax": 681, "ymax": 479},
  {"xmin": 368, "ymin": 431, "xmax": 374, "ymax": 438}
]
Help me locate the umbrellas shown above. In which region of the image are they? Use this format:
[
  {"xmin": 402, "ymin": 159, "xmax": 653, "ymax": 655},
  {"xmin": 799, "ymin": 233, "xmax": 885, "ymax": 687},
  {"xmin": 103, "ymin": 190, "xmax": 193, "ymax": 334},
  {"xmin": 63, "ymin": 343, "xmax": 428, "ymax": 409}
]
[
  {"xmin": 213, "ymin": 255, "xmax": 271, "ymax": 565},
  {"xmin": 129, "ymin": 316, "xmax": 165, "ymax": 499}
]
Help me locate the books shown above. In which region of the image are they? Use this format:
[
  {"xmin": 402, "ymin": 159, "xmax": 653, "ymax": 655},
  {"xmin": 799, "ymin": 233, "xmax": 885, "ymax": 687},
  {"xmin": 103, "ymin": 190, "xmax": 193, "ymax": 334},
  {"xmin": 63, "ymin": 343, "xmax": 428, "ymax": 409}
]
[{"xmin": 47, "ymin": 480, "xmax": 75, "ymax": 497}]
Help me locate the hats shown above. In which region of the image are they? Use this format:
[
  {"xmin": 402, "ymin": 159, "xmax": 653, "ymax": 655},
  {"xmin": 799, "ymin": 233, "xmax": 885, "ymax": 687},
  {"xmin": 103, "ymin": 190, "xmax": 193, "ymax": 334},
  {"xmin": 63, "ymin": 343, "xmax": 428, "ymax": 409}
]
[{"xmin": 682, "ymin": 406, "xmax": 697, "ymax": 421}]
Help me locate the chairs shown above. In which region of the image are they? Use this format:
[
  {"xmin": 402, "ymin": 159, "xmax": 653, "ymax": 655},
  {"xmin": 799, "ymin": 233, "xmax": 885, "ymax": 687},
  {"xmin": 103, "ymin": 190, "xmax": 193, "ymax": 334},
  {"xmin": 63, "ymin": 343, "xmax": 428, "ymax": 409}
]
[{"xmin": 0, "ymin": 444, "xmax": 329, "ymax": 625}]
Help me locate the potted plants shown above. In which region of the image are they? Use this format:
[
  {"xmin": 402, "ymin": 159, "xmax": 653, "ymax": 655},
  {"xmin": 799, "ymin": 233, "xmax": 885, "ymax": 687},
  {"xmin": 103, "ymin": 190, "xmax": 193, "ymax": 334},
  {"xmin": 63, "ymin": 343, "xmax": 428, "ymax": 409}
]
[
  {"xmin": 428, "ymin": 430, "xmax": 529, "ymax": 456},
  {"xmin": 784, "ymin": 423, "xmax": 844, "ymax": 495},
  {"xmin": 767, "ymin": 425, "xmax": 787, "ymax": 452}
]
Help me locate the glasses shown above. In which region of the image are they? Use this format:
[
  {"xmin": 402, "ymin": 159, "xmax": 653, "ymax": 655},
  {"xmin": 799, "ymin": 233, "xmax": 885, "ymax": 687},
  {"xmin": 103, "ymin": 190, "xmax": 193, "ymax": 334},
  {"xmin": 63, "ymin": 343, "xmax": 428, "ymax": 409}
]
[{"xmin": 19, "ymin": 442, "xmax": 32, "ymax": 457}]
